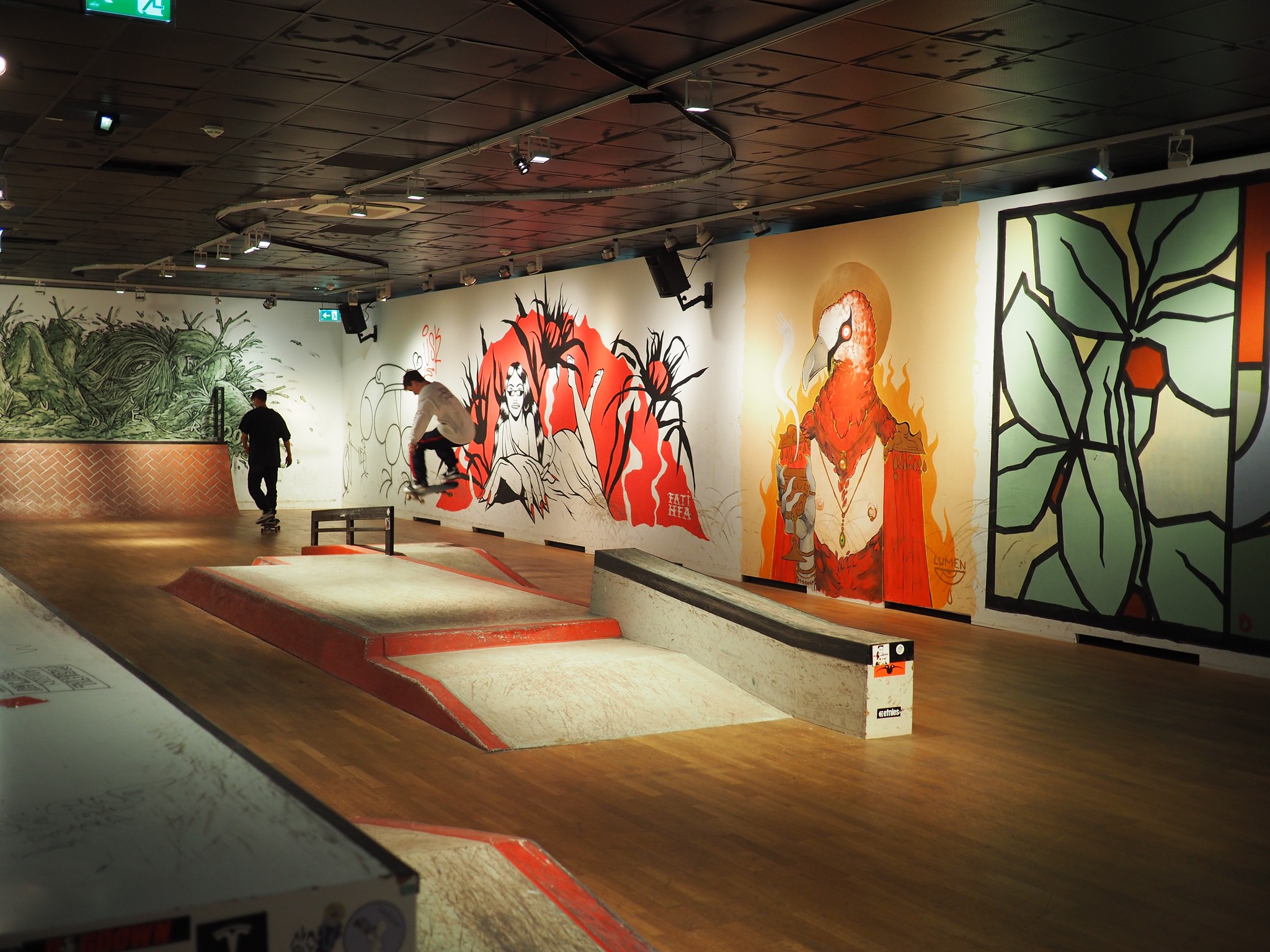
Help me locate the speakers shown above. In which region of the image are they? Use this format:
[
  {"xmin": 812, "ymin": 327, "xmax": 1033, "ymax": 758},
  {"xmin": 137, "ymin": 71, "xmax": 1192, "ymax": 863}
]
[
  {"xmin": 644, "ymin": 250, "xmax": 691, "ymax": 298},
  {"xmin": 338, "ymin": 302, "xmax": 367, "ymax": 334}
]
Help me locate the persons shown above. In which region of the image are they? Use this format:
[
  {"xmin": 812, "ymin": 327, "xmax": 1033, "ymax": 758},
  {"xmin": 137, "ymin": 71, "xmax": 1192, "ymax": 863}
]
[
  {"xmin": 238, "ymin": 389, "xmax": 292, "ymax": 523},
  {"xmin": 402, "ymin": 369, "xmax": 476, "ymax": 490}
]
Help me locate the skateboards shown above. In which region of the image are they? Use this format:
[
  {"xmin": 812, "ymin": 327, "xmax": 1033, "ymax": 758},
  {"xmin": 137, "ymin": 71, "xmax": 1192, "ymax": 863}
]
[
  {"xmin": 403, "ymin": 480, "xmax": 460, "ymax": 504},
  {"xmin": 258, "ymin": 518, "xmax": 281, "ymax": 535}
]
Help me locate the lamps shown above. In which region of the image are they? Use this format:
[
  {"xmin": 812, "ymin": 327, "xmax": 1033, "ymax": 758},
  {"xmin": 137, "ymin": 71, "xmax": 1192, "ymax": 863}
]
[
  {"xmin": 1167, "ymin": 129, "xmax": 1196, "ymax": 168},
  {"xmin": 696, "ymin": 223, "xmax": 714, "ymax": 247},
  {"xmin": 508, "ymin": 135, "xmax": 529, "ymax": 174},
  {"xmin": 601, "ymin": 239, "xmax": 619, "ymax": 262},
  {"xmin": 460, "ymin": 269, "xmax": 477, "ymax": 286},
  {"xmin": 526, "ymin": 254, "xmax": 543, "ymax": 275},
  {"xmin": 215, "ymin": 294, "xmax": 277, "ymax": 310},
  {"xmin": 498, "ymin": 258, "xmax": 515, "ymax": 279},
  {"xmin": 1092, "ymin": 147, "xmax": 1115, "ymax": 182},
  {"xmin": 527, "ymin": 128, "xmax": 552, "ymax": 164},
  {"xmin": 683, "ymin": 68, "xmax": 714, "ymax": 112},
  {"xmin": 941, "ymin": 173, "xmax": 962, "ymax": 206},
  {"xmin": 752, "ymin": 212, "xmax": 771, "ymax": 237},
  {"xmin": 194, "ymin": 226, "xmax": 271, "ymax": 268},
  {"xmin": 407, "ymin": 169, "xmax": 427, "ymax": 200},
  {"xmin": 349, "ymin": 189, "xmax": 367, "ymax": 217},
  {"xmin": 346, "ymin": 274, "xmax": 434, "ymax": 306},
  {"xmin": 115, "ymin": 276, "xmax": 146, "ymax": 302},
  {"xmin": 160, "ymin": 258, "xmax": 176, "ymax": 277},
  {"xmin": 664, "ymin": 228, "xmax": 681, "ymax": 251}
]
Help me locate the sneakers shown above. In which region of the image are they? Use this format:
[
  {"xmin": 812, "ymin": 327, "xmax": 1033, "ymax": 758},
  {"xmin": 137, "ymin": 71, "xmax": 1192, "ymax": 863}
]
[
  {"xmin": 403, "ymin": 479, "xmax": 430, "ymax": 494},
  {"xmin": 438, "ymin": 467, "xmax": 461, "ymax": 480},
  {"xmin": 256, "ymin": 509, "xmax": 276, "ymax": 524}
]
